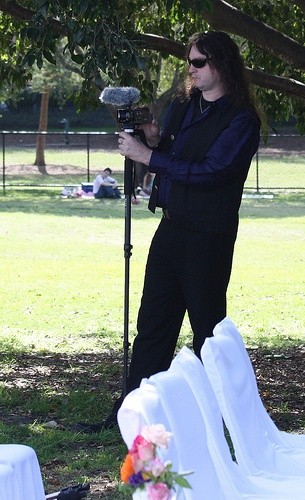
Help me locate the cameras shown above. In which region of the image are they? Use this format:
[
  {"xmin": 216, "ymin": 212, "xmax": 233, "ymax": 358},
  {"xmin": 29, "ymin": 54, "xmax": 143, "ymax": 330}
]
[{"xmin": 117, "ymin": 108, "xmax": 153, "ymax": 125}]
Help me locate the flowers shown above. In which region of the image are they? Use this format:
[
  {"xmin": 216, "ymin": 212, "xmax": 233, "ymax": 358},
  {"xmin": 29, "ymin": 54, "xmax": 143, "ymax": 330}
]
[{"xmin": 112, "ymin": 423, "xmax": 195, "ymax": 500}]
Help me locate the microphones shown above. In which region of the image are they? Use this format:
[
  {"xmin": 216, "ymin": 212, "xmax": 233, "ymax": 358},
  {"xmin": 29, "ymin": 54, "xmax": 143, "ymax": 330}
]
[{"xmin": 100, "ymin": 87, "xmax": 140, "ymax": 106}]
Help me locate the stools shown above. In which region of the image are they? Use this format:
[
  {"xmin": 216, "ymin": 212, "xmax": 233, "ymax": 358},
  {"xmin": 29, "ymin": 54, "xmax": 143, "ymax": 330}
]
[{"xmin": 0, "ymin": 443, "xmax": 45, "ymax": 499}]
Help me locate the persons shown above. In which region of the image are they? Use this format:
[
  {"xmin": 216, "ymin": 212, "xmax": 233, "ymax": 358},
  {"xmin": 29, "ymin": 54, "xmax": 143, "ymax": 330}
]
[
  {"xmin": 80, "ymin": 30, "xmax": 261, "ymax": 433},
  {"xmin": 93, "ymin": 168, "xmax": 121, "ymax": 198}
]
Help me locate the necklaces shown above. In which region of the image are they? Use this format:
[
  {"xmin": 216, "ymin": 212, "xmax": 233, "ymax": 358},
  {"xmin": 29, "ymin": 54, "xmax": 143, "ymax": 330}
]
[{"xmin": 198, "ymin": 92, "xmax": 210, "ymax": 113}]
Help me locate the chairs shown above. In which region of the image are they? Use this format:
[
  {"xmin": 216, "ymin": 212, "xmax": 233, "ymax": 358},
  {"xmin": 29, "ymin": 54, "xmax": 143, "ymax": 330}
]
[{"xmin": 117, "ymin": 317, "xmax": 305, "ymax": 500}]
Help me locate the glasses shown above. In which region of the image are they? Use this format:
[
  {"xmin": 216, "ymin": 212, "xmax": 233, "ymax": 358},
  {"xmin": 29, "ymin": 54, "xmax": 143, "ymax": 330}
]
[{"xmin": 187, "ymin": 56, "xmax": 211, "ymax": 68}]
